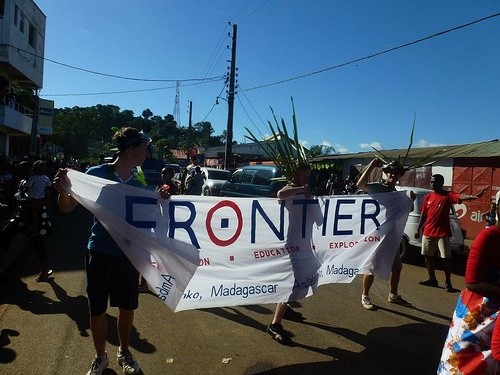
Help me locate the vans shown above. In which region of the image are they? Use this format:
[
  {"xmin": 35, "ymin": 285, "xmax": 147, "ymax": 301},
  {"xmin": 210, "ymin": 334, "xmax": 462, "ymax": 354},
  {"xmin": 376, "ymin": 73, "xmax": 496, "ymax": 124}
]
[{"xmin": 219, "ymin": 165, "xmax": 286, "ymax": 197}]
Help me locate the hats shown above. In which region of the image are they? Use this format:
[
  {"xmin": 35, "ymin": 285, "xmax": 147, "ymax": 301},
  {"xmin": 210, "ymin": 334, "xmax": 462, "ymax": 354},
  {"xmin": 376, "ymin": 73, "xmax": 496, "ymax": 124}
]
[{"xmin": 381, "ymin": 161, "xmax": 406, "ymax": 172}]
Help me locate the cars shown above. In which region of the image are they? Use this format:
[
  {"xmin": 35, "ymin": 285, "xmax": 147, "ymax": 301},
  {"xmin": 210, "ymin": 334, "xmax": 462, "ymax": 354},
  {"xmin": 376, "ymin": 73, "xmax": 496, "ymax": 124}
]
[
  {"xmin": 342, "ymin": 186, "xmax": 465, "ymax": 263},
  {"xmin": 161, "ymin": 164, "xmax": 233, "ymax": 196}
]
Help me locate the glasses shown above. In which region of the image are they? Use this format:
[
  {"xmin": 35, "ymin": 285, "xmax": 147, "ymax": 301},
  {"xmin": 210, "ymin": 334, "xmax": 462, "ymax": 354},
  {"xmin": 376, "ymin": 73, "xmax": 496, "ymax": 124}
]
[{"xmin": 126, "ymin": 129, "xmax": 152, "ymax": 146}]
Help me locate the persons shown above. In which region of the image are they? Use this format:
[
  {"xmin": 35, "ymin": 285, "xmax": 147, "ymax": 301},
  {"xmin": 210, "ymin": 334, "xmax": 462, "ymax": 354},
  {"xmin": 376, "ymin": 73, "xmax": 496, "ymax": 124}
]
[
  {"xmin": 267, "ymin": 165, "xmax": 324, "ymax": 345},
  {"xmin": 52, "ymin": 126, "xmax": 171, "ymax": 375},
  {"xmin": 355, "ymin": 158, "xmax": 412, "ymax": 310},
  {"xmin": 414, "ymin": 175, "xmax": 490, "ymax": 292},
  {"xmin": 437, "ymin": 190, "xmax": 500, "ymax": 375},
  {"xmin": 0, "ymin": 151, "xmax": 355, "ymax": 292}
]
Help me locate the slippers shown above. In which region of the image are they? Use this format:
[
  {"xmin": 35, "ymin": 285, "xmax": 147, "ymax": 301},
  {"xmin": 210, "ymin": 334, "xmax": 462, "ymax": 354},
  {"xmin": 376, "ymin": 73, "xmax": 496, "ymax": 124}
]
[{"xmin": 36, "ymin": 269, "xmax": 53, "ymax": 282}]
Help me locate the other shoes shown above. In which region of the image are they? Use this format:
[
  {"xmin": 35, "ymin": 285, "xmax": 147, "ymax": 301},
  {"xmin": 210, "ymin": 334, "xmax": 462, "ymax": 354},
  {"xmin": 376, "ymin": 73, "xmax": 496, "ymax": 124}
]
[
  {"xmin": 85, "ymin": 352, "xmax": 109, "ymax": 375},
  {"xmin": 268, "ymin": 324, "xmax": 292, "ymax": 346},
  {"xmin": 361, "ymin": 294, "xmax": 374, "ymax": 310},
  {"xmin": 419, "ymin": 280, "xmax": 438, "ymax": 288},
  {"xmin": 284, "ymin": 307, "xmax": 303, "ymax": 320},
  {"xmin": 387, "ymin": 292, "xmax": 412, "ymax": 307},
  {"xmin": 445, "ymin": 283, "xmax": 452, "ymax": 292},
  {"xmin": 116, "ymin": 347, "xmax": 140, "ymax": 375}
]
